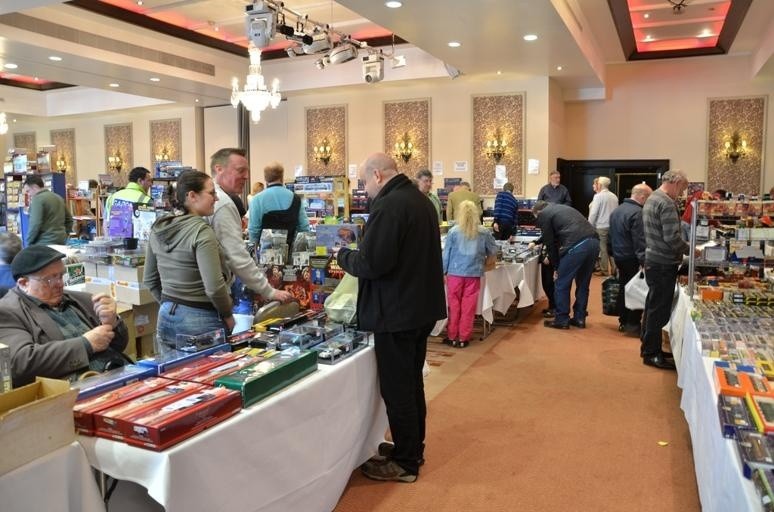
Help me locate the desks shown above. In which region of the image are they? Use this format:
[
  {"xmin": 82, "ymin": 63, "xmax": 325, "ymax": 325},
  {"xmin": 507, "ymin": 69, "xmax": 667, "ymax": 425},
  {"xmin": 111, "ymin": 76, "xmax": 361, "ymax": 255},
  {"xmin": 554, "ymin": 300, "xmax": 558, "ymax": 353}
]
[{"xmin": 667, "ymin": 278, "xmax": 773, "ymax": 512}]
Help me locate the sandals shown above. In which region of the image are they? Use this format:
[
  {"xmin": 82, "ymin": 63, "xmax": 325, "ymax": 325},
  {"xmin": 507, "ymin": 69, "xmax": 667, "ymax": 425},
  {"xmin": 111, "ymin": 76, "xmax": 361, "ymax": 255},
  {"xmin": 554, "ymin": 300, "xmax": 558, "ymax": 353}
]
[{"xmin": 593, "ymin": 264, "xmax": 601, "ymax": 272}]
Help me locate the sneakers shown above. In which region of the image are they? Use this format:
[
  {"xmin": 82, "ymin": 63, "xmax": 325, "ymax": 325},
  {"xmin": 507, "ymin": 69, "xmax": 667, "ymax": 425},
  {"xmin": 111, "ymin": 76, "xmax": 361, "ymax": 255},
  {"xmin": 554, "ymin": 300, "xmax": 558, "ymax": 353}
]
[
  {"xmin": 378, "ymin": 443, "xmax": 424, "ymax": 467},
  {"xmin": 458, "ymin": 340, "xmax": 469, "ymax": 348},
  {"xmin": 593, "ymin": 271, "xmax": 609, "ymax": 276},
  {"xmin": 547, "ymin": 310, "xmax": 556, "ymax": 317},
  {"xmin": 360, "ymin": 458, "xmax": 418, "ymax": 484},
  {"xmin": 442, "ymin": 337, "xmax": 457, "ymax": 347}
]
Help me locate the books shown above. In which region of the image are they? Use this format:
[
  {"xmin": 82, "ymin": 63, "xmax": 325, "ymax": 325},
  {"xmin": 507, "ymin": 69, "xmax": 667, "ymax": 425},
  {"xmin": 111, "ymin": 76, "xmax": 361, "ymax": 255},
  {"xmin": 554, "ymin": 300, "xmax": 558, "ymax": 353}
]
[{"xmin": 0, "ymin": 343, "xmax": 13, "ymax": 393}]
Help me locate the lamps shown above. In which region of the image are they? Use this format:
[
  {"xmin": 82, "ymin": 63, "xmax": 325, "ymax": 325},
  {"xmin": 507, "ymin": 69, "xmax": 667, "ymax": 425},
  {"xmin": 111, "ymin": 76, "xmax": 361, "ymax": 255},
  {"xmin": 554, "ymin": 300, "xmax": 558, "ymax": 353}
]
[{"xmin": 244, "ymin": 0, "xmax": 405, "ymax": 85}]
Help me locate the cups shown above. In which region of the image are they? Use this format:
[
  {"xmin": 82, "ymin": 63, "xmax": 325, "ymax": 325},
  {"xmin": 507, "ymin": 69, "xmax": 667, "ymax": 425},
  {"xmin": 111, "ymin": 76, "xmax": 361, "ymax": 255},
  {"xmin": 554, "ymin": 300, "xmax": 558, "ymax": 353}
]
[{"xmin": 123, "ymin": 238, "xmax": 138, "ymax": 250}]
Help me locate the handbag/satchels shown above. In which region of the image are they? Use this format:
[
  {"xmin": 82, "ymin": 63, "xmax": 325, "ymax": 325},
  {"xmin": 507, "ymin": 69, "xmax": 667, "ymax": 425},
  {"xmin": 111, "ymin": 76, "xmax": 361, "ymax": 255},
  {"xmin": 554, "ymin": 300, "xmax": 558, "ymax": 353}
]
[{"xmin": 602, "ymin": 268, "xmax": 621, "ymax": 316}]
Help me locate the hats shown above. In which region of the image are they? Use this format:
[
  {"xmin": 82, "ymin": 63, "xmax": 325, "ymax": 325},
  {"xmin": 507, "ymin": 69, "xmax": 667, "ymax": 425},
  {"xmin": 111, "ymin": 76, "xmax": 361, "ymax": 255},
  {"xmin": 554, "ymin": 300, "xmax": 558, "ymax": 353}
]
[{"xmin": 11, "ymin": 245, "xmax": 67, "ymax": 282}]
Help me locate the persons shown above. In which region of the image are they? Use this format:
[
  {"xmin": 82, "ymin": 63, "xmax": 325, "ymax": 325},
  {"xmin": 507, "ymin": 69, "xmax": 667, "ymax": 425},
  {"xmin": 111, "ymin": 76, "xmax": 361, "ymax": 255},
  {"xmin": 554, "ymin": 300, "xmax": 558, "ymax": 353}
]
[
  {"xmin": 441, "ymin": 200, "xmax": 498, "ymax": 347},
  {"xmin": 533, "ymin": 202, "xmax": 599, "ymax": 329},
  {"xmin": 537, "ymin": 171, "xmax": 572, "ymax": 206},
  {"xmin": 0, "ymin": 244, "xmax": 137, "ymax": 389},
  {"xmin": 104, "ymin": 164, "xmax": 156, "ymax": 239},
  {"xmin": 609, "ymin": 183, "xmax": 653, "ymax": 337},
  {"xmin": 447, "ymin": 181, "xmax": 483, "ymax": 220},
  {"xmin": 638, "ymin": 169, "xmax": 700, "ymax": 370},
  {"xmin": 415, "ymin": 170, "xmax": 443, "ymax": 225},
  {"xmin": 332, "ymin": 152, "xmax": 449, "ymax": 484},
  {"xmin": 244, "ymin": 183, "xmax": 263, "ymax": 218},
  {"xmin": 250, "ymin": 165, "xmax": 310, "ymax": 241},
  {"xmin": 0, "ymin": 230, "xmax": 21, "ymax": 294},
  {"xmin": 588, "ymin": 177, "xmax": 618, "ymax": 275},
  {"xmin": 494, "ymin": 183, "xmax": 518, "ymax": 239},
  {"xmin": 144, "ymin": 170, "xmax": 235, "ymax": 352},
  {"xmin": 527, "ymin": 234, "xmax": 560, "ymax": 318},
  {"xmin": 203, "ymin": 148, "xmax": 292, "ymax": 335},
  {"xmin": 23, "ymin": 173, "xmax": 73, "ymax": 249}
]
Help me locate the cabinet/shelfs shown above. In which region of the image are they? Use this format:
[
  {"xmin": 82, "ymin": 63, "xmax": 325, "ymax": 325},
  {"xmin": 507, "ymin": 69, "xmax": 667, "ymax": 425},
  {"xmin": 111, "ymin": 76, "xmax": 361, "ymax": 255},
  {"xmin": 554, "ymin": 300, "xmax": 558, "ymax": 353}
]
[
  {"xmin": 0, "ymin": 172, "xmax": 112, "ymax": 240},
  {"xmin": 293, "ymin": 178, "xmax": 349, "ymax": 236}
]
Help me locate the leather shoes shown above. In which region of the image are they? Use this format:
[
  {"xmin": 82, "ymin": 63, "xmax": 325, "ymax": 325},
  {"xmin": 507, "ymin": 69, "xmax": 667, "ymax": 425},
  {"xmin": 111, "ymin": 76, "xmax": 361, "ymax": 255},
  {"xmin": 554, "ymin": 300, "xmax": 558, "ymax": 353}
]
[
  {"xmin": 643, "ymin": 353, "xmax": 676, "ymax": 370},
  {"xmin": 641, "ymin": 351, "xmax": 673, "ymax": 357},
  {"xmin": 619, "ymin": 324, "xmax": 626, "ymax": 333},
  {"xmin": 569, "ymin": 318, "xmax": 585, "ymax": 329},
  {"xmin": 625, "ymin": 325, "xmax": 643, "ymax": 338},
  {"xmin": 544, "ymin": 320, "xmax": 569, "ymax": 330}
]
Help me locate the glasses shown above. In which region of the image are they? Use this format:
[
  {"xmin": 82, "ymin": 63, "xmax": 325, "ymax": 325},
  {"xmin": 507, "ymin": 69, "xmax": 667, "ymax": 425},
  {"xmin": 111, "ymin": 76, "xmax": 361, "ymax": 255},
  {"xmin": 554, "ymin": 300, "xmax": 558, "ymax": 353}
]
[
  {"xmin": 185, "ymin": 192, "xmax": 217, "ymax": 199},
  {"xmin": 22, "ymin": 273, "xmax": 66, "ymax": 289}
]
